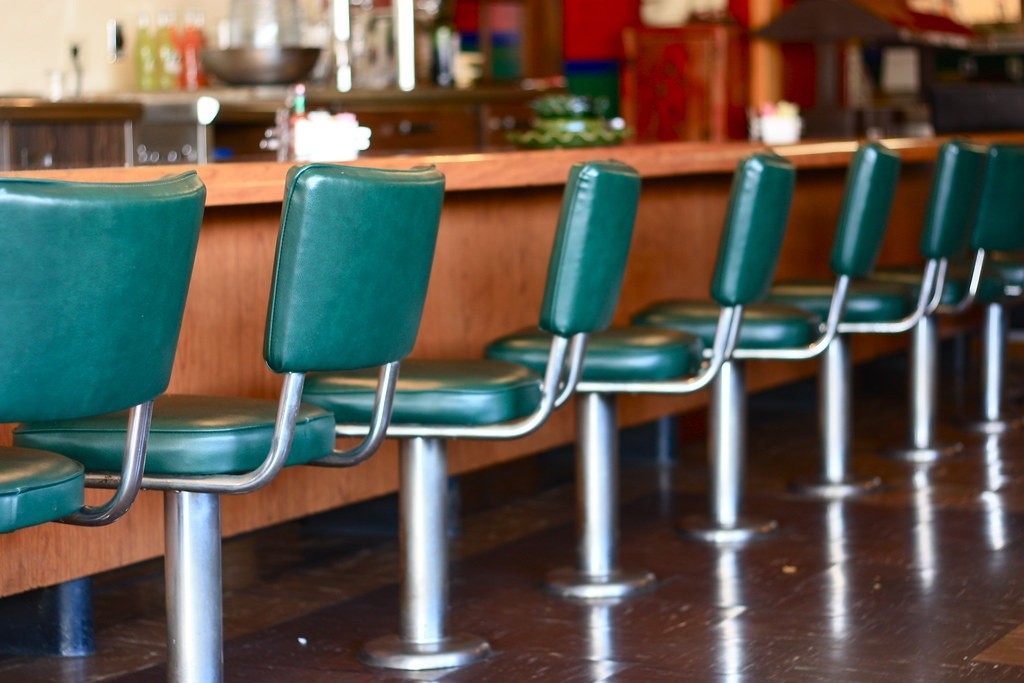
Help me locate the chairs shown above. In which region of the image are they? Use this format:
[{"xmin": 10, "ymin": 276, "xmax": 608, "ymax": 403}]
[
  {"xmin": 771, "ymin": 135, "xmax": 987, "ymax": 462},
  {"xmin": 0, "ymin": 171, "xmax": 206, "ymax": 529},
  {"xmin": 485, "ymin": 156, "xmax": 796, "ymax": 603},
  {"xmin": 292, "ymin": 161, "xmax": 638, "ymax": 671},
  {"xmin": 767, "ymin": 134, "xmax": 996, "ymax": 501},
  {"xmin": 635, "ymin": 140, "xmax": 912, "ymax": 544},
  {"xmin": 944, "ymin": 142, "xmax": 1023, "ymax": 437},
  {"xmin": 12, "ymin": 163, "xmax": 446, "ymax": 683}
]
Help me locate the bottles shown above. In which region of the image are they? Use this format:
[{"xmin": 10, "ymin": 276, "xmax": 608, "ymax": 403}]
[{"xmin": 285, "ymin": 94, "xmax": 310, "ymax": 162}]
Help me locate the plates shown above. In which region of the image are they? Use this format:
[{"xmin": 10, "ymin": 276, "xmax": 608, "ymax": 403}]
[{"xmin": 506, "ymin": 124, "xmax": 633, "ymax": 147}]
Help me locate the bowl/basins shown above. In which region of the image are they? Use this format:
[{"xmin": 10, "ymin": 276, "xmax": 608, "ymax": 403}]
[
  {"xmin": 198, "ymin": 45, "xmax": 321, "ymax": 83},
  {"xmin": 526, "ymin": 93, "xmax": 610, "ymax": 132}
]
